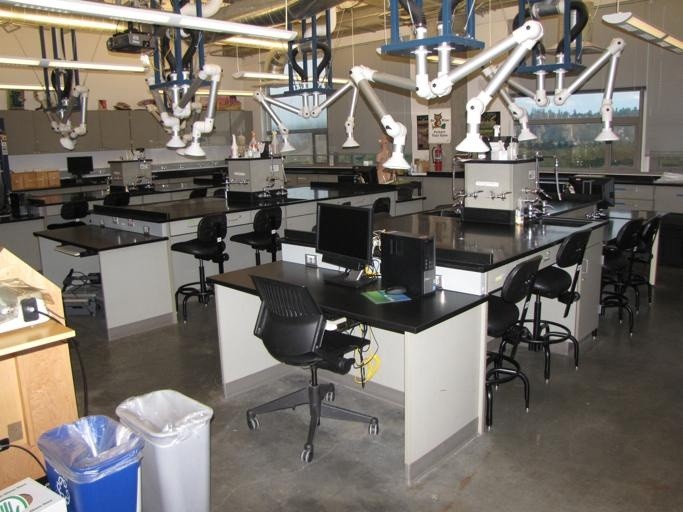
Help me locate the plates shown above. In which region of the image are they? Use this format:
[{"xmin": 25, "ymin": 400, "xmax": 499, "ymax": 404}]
[{"xmin": 112, "ymin": 106, "xmax": 131, "ymax": 110}]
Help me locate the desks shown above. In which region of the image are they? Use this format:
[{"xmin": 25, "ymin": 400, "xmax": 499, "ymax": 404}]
[{"xmin": 30, "ymin": 224, "xmax": 175, "ymax": 343}]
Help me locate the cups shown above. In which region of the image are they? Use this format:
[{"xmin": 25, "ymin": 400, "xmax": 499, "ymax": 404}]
[
  {"xmin": 407, "ymin": 164, "xmax": 416, "ymax": 174},
  {"xmin": 435, "ymin": 275, "xmax": 441, "ymax": 290},
  {"xmin": 97, "ymin": 100, "xmax": 106, "ymax": 109}
]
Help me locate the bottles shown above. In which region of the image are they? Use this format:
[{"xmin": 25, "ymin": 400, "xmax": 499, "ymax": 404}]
[
  {"xmin": 418, "ymin": 160, "xmax": 424, "ymax": 173},
  {"xmin": 514, "ymin": 197, "xmax": 525, "ymax": 225},
  {"xmin": 477, "ymin": 125, "xmax": 519, "ymax": 160},
  {"xmin": 119, "ymin": 144, "xmax": 144, "ymax": 161},
  {"xmin": 231, "ymin": 130, "xmax": 280, "ymax": 158}
]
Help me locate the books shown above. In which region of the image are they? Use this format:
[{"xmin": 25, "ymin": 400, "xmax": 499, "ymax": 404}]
[{"xmin": 363, "ymin": 287, "xmax": 412, "ymax": 305}]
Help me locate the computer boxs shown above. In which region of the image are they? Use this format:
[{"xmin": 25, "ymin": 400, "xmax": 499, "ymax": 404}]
[{"xmin": 380, "ymin": 230, "xmax": 437, "ymax": 297}]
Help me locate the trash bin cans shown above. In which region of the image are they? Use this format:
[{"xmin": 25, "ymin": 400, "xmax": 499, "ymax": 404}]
[
  {"xmin": 37, "ymin": 390, "xmax": 214, "ymax": 512},
  {"xmin": 660, "ymin": 213, "xmax": 683, "ymax": 265}
]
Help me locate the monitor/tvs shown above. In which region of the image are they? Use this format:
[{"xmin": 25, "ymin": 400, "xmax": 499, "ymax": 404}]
[{"xmin": 315, "ymin": 201, "xmax": 378, "ymax": 291}]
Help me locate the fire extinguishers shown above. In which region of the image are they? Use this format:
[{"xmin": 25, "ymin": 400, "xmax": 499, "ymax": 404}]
[{"xmin": 432, "ymin": 145, "xmax": 443, "ymax": 173}]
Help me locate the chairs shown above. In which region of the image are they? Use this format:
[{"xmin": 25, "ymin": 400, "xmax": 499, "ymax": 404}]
[{"xmin": 51, "ymin": 196, "xmax": 89, "ymax": 230}]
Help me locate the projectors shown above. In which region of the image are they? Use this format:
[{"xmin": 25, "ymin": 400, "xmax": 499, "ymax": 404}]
[{"xmin": 105, "ymin": 30, "xmax": 157, "ymax": 53}]
